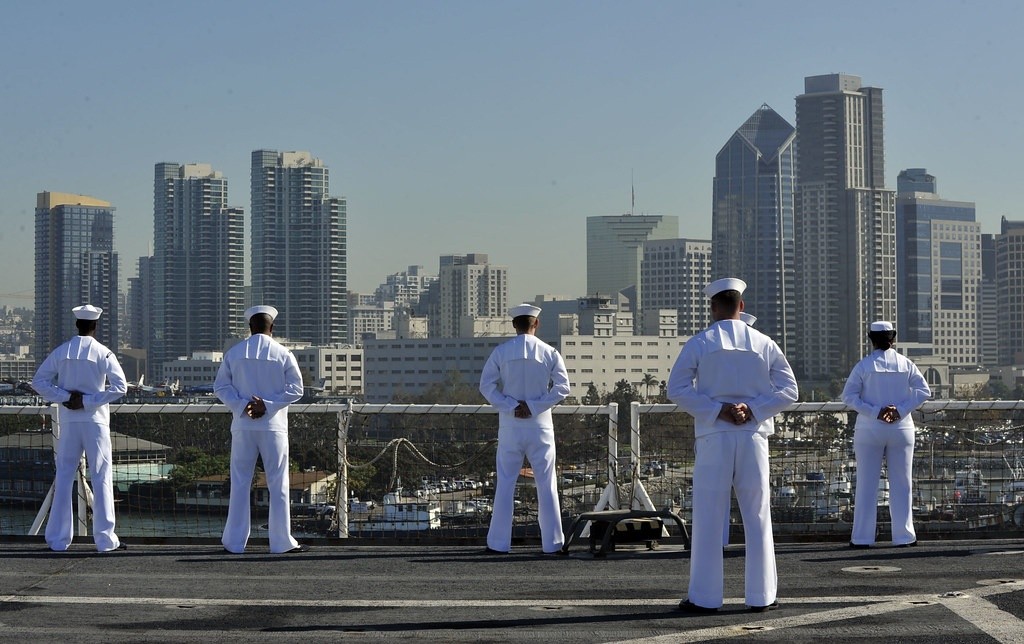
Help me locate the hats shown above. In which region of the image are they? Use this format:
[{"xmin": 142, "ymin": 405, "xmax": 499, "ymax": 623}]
[
  {"xmin": 867, "ymin": 322, "xmax": 896, "ymax": 341},
  {"xmin": 244, "ymin": 305, "xmax": 278, "ymax": 321},
  {"xmin": 739, "ymin": 311, "xmax": 757, "ymax": 327},
  {"xmin": 702, "ymin": 278, "xmax": 747, "ymax": 298},
  {"xmin": 508, "ymin": 304, "xmax": 543, "ymax": 320},
  {"xmin": 72, "ymin": 305, "xmax": 103, "ymax": 320}
]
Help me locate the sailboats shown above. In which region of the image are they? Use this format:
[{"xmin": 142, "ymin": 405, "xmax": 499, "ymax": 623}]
[{"xmin": 913, "ymin": 442, "xmax": 956, "ymax": 484}]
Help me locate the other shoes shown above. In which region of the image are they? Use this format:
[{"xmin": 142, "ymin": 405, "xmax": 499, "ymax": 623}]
[
  {"xmin": 292, "ymin": 543, "xmax": 309, "ymax": 553},
  {"xmin": 850, "ymin": 540, "xmax": 870, "ymax": 549},
  {"xmin": 678, "ymin": 594, "xmax": 717, "ymax": 612},
  {"xmin": 486, "ymin": 544, "xmax": 508, "ymax": 555},
  {"xmin": 901, "ymin": 540, "xmax": 917, "ymax": 548},
  {"xmin": 114, "ymin": 542, "xmax": 126, "ymax": 550},
  {"xmin": 754, "ymin": 599, "xmax": 779, "ymax": 613}
]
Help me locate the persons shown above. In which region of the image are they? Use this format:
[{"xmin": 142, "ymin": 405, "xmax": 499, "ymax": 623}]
[
  {"xmin": 479, "ymin": 304, "xmax": 565, "ymax": 554},
  {"xmin": 841, "ymin": 321, "xmax": 931, "ymax": 548},
  {"xmin": 668, "ymin": 278, "xmax": 798, "ymax": 612},
  {"xmin": 213, "ymin": 305, "xmax": 311, "ymax": 553},
  {"xmin": 32, "ymin": 305, "xmax": 127, "ymax": 552}
]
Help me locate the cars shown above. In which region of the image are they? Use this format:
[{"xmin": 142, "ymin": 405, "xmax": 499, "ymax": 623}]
[{"xmin": 397, "ymin": 460, "xmax": 668, "ymax": 498}]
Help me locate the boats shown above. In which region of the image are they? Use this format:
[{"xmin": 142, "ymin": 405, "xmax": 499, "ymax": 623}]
[
  {"xmin": 440, "ymin": 501, "xmax": 492, "ymax": 521},
  {"xmin": 348, "ymin": 489, "xmax": 442, "ymax": 536},
  {"xmin": 471, "ymin": 498, "xmax": 490, "ymax": 508},
  {"xmin": 952, "ymin": 469, "xmax": 989, "ymax": 491},
  {"xmin": 996, "ymin": 491, "xmax": 1024, "ymax": 506},
  {"xmin": 1001, "ymin": 453, "xmax": 1024, "ymax": 491},
  {"xmin": 770, "ymin": 459, "xmax": 923, "ymax": 516},
  {"xmin": 348, "ymin": 491, "xmax": 378, "ymax": 513}
]
